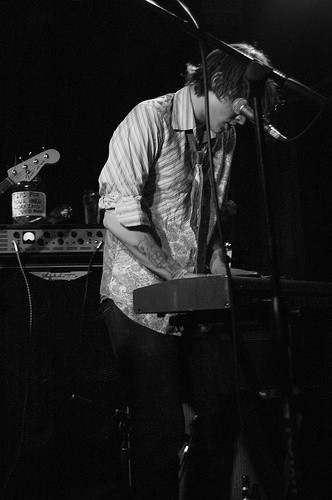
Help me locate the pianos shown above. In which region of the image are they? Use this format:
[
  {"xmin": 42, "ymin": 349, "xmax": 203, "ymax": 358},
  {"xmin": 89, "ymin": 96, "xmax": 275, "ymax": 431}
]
[{"xmin": 132, "ymin": 276, "xmax": 332, "ymax": 319}]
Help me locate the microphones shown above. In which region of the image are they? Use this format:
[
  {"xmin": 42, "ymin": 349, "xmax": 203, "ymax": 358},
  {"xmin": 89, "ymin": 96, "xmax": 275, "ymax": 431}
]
[{"xmin": 232, "ymin": 97, "xmax": 288, "ymax": 145}]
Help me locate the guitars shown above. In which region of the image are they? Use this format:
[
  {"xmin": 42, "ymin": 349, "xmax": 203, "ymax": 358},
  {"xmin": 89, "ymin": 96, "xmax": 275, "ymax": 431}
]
[{"xmin": 1, "ymin": 144, "xmax": 61, "ymax": 198}]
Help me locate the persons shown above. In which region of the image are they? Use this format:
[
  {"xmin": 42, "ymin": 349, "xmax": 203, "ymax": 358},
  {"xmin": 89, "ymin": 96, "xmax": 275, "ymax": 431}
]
[{"xmin": 85, "ymin": 44, "xmax": 283, "ymax": 500}]
